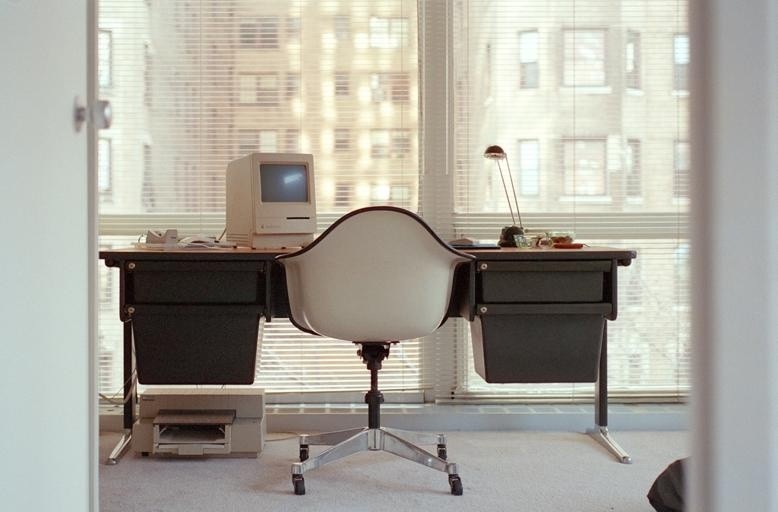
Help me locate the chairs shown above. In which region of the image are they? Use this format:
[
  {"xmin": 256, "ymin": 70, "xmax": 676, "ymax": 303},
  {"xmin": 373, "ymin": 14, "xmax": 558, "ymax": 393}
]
[{"xmin": 275, "ymin": 206, "xmax": 478, "ymax": 494}]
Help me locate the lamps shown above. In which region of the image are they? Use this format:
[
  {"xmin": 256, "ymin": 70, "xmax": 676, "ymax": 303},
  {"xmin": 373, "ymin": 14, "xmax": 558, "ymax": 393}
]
[{"xmin": 483, "ymin": 145, "xmax": 524, "ymax": 233}]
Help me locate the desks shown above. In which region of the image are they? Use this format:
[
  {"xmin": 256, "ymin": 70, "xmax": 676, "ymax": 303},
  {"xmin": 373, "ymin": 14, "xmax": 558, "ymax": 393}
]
[{"xmin": 99, "ymin": 242, "xmax": 637, "ymax": 465}]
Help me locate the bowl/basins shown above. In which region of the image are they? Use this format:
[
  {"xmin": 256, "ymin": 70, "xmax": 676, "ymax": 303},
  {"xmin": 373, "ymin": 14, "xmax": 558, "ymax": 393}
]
[{"xmin": 513, "ymin": 232, "xmax": 576, "ymax": 249}]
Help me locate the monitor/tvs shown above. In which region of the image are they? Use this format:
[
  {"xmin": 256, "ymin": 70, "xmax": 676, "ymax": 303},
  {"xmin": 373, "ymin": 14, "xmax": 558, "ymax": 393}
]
[{"xmin": 226, "ymin": 151, "xmax": 317, "ymax": 248}]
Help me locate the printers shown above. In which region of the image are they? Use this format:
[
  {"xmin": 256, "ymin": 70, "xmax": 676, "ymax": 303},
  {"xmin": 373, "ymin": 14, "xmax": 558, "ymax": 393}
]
[{"xmin": 133, "ymin": 384, "xmax": 265, "ymax": 459}]
